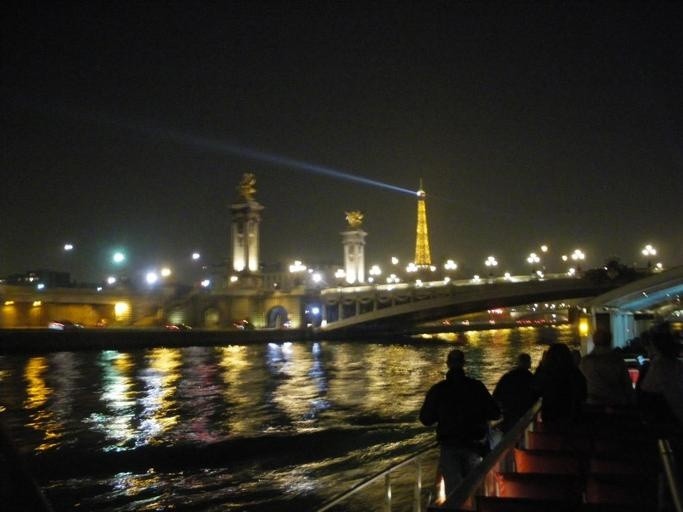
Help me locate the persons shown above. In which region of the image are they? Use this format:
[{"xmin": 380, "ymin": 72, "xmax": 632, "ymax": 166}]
[
  {"xmin": 419, "ymin": 348, "xmax": 502, "ymax": 512},
  {"xmin": 492, "ymin": 350, "xmax": 533, "ymax": 431},
  {"xmin": 532, "ymin": 322, "xmax": 682, "ymax": 434}
]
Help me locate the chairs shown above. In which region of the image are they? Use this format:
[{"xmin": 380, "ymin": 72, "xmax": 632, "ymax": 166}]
[{"xmin": 473, "ymin": 411, "xmax": 659, "ymax": 511}]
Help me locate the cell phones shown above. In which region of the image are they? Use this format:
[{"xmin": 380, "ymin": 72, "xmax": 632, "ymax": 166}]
[{"xmin": 637, "ymin": 355, "xmax": 644, "ymax": 364}]
